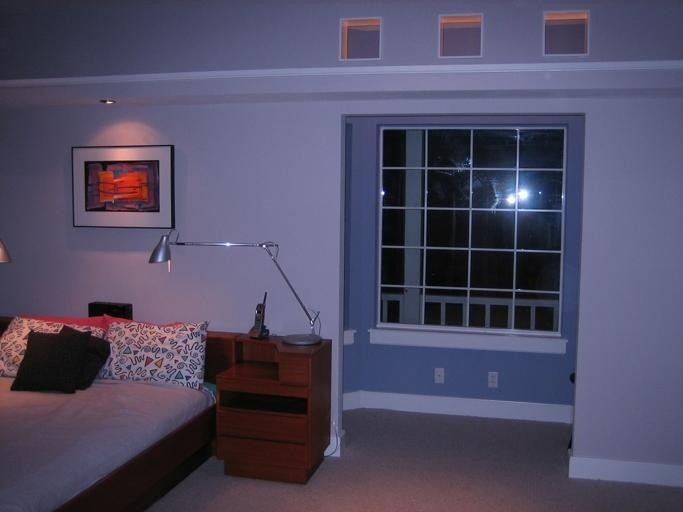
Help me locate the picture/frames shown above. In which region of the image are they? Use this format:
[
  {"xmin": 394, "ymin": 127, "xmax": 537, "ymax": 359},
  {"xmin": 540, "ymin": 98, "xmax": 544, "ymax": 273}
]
[{"xmin": 70, "ymin": 145, "xmax": 175, "ymax": 229}]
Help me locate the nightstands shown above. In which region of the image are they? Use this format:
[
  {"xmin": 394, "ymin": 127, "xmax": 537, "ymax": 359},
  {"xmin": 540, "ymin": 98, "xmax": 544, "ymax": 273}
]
[{"xmin": 216, "ymin": 335, "xmax": 332, "ymax": 484}]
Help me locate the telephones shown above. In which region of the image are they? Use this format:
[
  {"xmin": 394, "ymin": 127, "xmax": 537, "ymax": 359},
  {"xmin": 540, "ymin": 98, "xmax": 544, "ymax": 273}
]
[{"xmin": 248, "ymin": 292, "xmax": 269, "ymax": 339}]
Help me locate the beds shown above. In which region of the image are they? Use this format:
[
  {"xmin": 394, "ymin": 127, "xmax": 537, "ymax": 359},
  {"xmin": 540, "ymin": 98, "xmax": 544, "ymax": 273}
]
[{"xmin": 0, "ymin": 314, "xmax": 244, "ymax": 512}]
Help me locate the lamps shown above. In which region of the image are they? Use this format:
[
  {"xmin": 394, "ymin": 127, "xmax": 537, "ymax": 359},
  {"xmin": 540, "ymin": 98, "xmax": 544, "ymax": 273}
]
[
  {"xmin": 149, "ymin": 229, "xmax": 321, "ymax": 345},
  {"xmin": 0, "ymin": 239, "xmax": 11, "ymax": 262}
]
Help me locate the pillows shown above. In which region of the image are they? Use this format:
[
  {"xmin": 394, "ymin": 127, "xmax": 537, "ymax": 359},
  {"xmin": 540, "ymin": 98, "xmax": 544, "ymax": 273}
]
[{"xmin": 1, "ymin": 313, "xmax": 211, "ymax": 394}]
